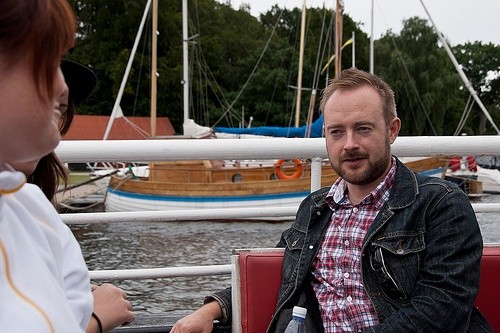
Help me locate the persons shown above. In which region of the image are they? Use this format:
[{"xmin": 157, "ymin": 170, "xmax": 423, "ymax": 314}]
[
  {"xmin": 0, "ymin": 0, "xmax": 94, "ymax": 333},
  {"xmin": 27, "ymin": 154, "xmax": 135, "ymax": 333},
  {"xmin": 169, "ymin": 70, "xmax": 491, "ymax": 333},
  {"xmin": 449, "ymin": 157, "xmax": 460, "ymax": 172},
  {"xmin": 466, "ymin": 156, "xmax": 477, "ymax": 172}
]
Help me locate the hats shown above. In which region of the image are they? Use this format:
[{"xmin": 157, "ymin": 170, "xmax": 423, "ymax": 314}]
[{"xmin": 59, "ymin": 57, "xmax": 97, "ymax": 103}]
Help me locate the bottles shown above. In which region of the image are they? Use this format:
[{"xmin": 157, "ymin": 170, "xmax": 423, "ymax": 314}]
[{"xmin": 284, "ymin": 306, "xmax": 307, "ymax": 333}]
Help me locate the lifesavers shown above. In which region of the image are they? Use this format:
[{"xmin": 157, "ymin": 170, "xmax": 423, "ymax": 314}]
[{"xmin": 275, "ymin": 159, "xmax": 303, "ymax": 179}]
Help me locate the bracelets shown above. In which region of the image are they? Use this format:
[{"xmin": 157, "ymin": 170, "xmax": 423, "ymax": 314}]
[{"xmin": 92, "ymin": 313, "xmax": 103, "ymax": 333}]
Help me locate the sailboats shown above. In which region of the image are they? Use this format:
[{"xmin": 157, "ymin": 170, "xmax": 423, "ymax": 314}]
[{"xmin": 53, "ymin": 0, "xmax": 499, "ymax": 222}]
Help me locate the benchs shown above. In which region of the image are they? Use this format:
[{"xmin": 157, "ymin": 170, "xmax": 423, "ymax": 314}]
[{"xmin": 231, "ymin": 243, "xmax": 500, "ymax": 333}]
